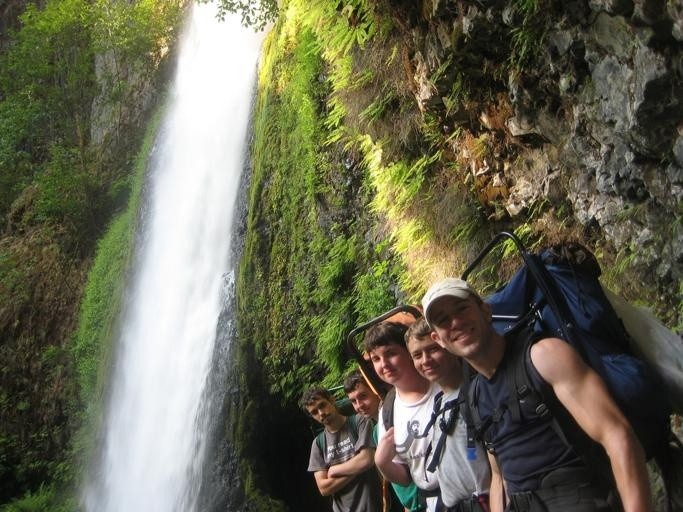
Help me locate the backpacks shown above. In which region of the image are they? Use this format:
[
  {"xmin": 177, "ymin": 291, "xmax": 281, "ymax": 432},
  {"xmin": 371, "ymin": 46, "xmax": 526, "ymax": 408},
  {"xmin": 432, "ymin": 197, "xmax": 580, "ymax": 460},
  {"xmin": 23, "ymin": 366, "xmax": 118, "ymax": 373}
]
[{"xmin": 485, "ymin": 242, "xmax": 674, "ymax": 462}]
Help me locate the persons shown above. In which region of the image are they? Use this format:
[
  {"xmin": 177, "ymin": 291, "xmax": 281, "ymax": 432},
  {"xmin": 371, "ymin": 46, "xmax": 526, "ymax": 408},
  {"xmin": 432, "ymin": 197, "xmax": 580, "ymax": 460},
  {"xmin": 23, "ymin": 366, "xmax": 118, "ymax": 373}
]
[
  {"xmin": 422, "ymin": 276, "xmax": 653, "ymax": 511},
  {"xmin": 362, "ymin": 321, "xmax": 442, "ymax": 511},
  {"xmin": 341, "ymin": 369, "xmax": 427, "ymax": 511},
  {"xmin": 403, "ymin": 315, "xmax": 491, "ymax": 511},
  {"xmin": 301, "ymin": 384, "xmax": 383, "ymax": 511}
]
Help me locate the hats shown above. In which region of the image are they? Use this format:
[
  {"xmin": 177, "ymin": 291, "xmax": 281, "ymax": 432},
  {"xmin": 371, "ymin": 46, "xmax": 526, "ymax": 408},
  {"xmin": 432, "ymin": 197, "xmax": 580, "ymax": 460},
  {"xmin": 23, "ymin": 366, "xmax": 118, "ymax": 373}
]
[{"xmin": 421, "ymin": 278, "xmax": 483, "ymax": 330}]
